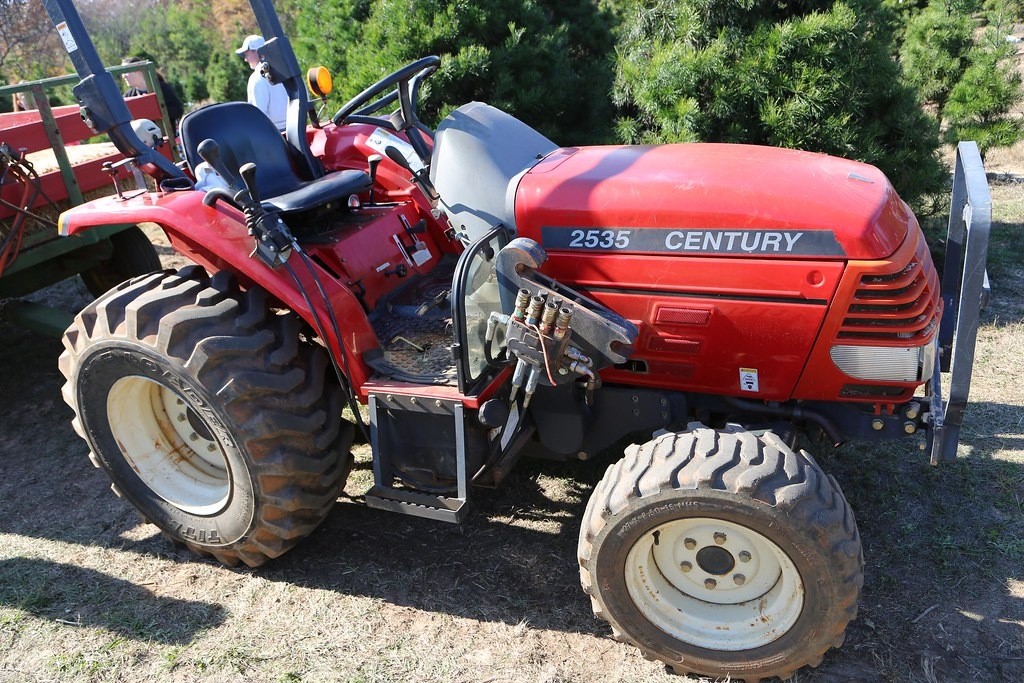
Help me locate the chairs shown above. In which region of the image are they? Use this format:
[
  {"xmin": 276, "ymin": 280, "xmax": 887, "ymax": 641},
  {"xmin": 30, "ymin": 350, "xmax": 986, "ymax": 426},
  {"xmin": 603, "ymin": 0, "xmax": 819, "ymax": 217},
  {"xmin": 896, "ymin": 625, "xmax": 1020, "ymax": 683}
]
[{"xmin": 181, "ymin": 101, "xmax": 374, "ymax": 217}]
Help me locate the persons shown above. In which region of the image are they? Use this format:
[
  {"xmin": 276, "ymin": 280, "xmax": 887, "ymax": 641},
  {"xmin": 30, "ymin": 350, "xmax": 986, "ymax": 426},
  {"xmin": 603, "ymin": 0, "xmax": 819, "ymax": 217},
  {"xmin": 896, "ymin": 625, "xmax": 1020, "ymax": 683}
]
[
  {"xmin": 120, "ymin": 54, "xmax": 184, "ymax": 136},
  {"xmin": 234, "ymin": 33, "xmax": 294, "ymax": 137},
  {"xmin": 9, "ymin": 76, "xmax": 46, "ymax": 112}
]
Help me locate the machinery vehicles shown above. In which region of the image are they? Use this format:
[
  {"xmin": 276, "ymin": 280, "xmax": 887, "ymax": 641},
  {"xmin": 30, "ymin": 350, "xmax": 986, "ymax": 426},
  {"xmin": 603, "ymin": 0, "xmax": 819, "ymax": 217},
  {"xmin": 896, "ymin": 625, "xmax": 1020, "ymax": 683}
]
[{"xmin": 39, "ymin": 1, "xmax": 996, "ymax": 682}]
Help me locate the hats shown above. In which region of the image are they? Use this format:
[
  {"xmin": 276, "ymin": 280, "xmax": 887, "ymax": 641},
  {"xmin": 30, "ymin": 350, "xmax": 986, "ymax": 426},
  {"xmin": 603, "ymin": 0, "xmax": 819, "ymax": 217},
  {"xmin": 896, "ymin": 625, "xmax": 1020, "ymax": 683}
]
[{"xmin": 235, "ymin": 35, "xmax": 265, "ymax": 53}]
